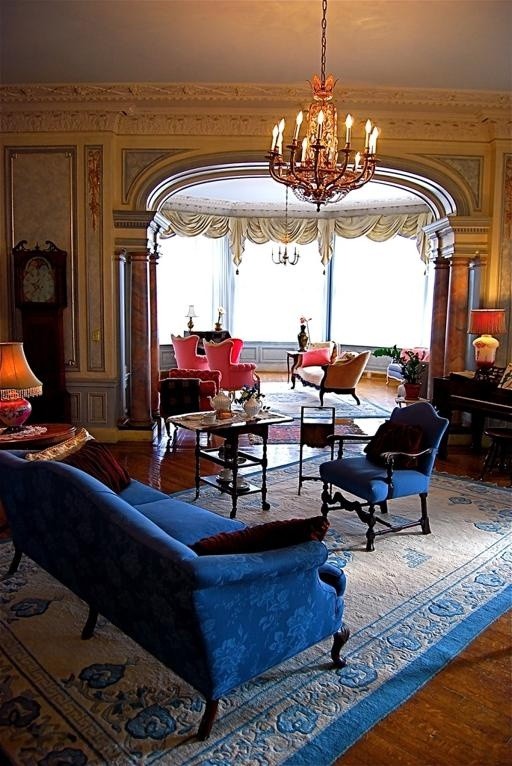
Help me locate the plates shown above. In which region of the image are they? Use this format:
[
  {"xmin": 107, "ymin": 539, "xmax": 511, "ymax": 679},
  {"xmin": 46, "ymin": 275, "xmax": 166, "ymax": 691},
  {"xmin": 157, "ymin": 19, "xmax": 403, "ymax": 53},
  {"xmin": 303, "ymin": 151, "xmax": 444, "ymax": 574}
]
[
  {"xmin": 216, "ymin": 468, "xmax": 250, "ymax": 490},
  {"xmin": 200, "ymin": 419, "xmax": 219, "ymax": 426}
]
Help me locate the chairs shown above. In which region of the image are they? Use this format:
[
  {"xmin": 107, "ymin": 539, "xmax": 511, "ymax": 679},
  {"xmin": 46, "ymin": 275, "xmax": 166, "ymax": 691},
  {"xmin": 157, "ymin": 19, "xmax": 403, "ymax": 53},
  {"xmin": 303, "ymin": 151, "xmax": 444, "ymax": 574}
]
[
  {"xmin": 170, "ymin": 333, "xmax": 215, "ymax": 376},
  {"xmin": 313, "ymin": 398, "xmax": 451, "ymax": 554},
  {"xmin": 201, "ymin": 336, "xmax": 266, "ymax": 393},
  {"xmin": 298, "ymin": 403, "xmax": 337, "ymax": 498},
  {"xmin": 209, "ymin": 339, "xmax": 243, "ymax": 363}
]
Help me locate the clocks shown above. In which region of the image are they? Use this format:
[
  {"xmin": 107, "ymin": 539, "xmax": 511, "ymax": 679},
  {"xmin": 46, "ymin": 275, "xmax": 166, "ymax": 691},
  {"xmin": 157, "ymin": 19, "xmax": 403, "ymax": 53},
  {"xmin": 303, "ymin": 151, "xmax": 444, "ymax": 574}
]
[{"xmin": 9, "ymin": 238, "xmax": 80, "ymax": 427}]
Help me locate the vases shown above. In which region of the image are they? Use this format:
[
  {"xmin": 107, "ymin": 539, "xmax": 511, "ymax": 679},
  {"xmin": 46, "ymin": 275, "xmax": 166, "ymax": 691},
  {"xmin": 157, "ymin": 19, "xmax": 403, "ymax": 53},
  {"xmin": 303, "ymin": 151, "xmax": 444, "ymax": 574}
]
[
  {"xmin": 295, "ymin": 323, "xmax": 311, "ymax": 350},
  {"xmin": 214, "ymin": 319, "xmax": 225, "ymax": 333},
  {"xmin": 243, "ymin": 398, "xmax": 263, "ymax": 418}
]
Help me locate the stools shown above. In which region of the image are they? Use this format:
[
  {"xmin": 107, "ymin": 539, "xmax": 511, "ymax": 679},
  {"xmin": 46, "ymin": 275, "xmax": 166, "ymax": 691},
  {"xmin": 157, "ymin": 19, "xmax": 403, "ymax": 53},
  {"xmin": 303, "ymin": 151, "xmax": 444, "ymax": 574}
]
[{"xmin": 481, "ymin": 425, "xmax": 512, "ymax": 475}]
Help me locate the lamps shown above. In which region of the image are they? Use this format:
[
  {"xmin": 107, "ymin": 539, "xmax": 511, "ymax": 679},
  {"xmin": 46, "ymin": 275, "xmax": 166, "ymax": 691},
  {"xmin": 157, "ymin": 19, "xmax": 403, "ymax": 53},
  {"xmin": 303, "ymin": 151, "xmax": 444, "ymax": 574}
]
[
  {"xmin": 187, "ymin": 302, "xmax": 201, "ymax": 330},
  {"xmin": 263, "ymin": 4, "xmax": 383, "ymax": 214},
  {"xmin": 465, "ymin": 307, "xmax": 509, "ymax": 382},
  {"xmin": 269, "ymin": 234, "xmax": 305, "ymax": 269},
  {"xmin": 0, "ymin": 340, "xmax": 48, "ymax": 434}
]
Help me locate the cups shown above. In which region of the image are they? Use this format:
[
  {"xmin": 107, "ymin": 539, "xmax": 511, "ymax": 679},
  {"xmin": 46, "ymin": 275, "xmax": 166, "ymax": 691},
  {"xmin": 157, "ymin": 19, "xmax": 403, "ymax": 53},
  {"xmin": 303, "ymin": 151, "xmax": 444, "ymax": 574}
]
[{"xmin": 203, "ymin": 413, "xmax": 217, "ymax": 424}]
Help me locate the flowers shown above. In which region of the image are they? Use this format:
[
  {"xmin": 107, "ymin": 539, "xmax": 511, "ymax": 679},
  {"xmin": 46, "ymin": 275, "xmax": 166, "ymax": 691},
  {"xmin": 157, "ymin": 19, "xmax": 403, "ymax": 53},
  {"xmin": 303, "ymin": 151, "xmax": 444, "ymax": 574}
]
[{"xmin": 236, "ymin": 384, "xmax": 266, "ymax": 404}]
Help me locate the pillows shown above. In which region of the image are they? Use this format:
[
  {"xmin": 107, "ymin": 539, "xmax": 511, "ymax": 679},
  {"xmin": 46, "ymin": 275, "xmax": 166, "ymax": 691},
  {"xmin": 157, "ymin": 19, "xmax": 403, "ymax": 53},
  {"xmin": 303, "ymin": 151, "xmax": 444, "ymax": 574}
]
[
  {"xmin": 186, "ymin": 515, "xmax": 341, "ymax": 566},
  {"xmin": 23, "ymin": 429, "xmax": 94, "ymax": 462},
  {"xmin": 56, "ymin": 439, "xmax": 136, "ymax": 497},
  {"xmin": 300, "ymin": 347, "xmax": 333, "ymax": 370},
  {"xmin": 364, "ymin": 417, "xmax": 421, "ymax": 471},
  {"xmin": 307, "ymin": 342, "xmax": 335, "ymax": 357}
]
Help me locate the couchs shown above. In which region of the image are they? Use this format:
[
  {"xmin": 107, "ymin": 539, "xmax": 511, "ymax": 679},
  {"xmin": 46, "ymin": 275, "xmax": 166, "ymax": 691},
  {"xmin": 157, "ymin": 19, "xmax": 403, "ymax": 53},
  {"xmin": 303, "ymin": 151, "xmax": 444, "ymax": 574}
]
[
  {"xmin": 287, "ymin": 337, "xmax": 374, "ymax": 407},
  {"xmin": 0, "ymin": 436, "xmax": 356, "ymax": 746},
  {"xmin": 159, "ymin": 365, "xmax": 224, "ymax": 409},
  {"xmin": 384, "ymin": 347, "xmax": 428, "ymax": 391}
]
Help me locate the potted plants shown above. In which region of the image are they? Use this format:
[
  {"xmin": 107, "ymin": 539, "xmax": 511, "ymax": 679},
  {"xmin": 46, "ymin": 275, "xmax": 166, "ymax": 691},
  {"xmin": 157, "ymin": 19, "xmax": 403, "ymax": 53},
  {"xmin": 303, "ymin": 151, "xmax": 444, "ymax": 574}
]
[{"xmin": 371, "ymin": 344, "xmax": 429, "ymax": 399}]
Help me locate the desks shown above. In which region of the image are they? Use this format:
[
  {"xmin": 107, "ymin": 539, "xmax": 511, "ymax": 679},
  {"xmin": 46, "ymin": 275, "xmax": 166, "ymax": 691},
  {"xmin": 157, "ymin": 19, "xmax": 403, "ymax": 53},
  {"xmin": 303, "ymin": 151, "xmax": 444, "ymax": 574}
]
[
  {"xmin": 0, "ymin": 422, "xmax": 77, "ymax": 469},
  {"xmin": 285, "ymin": 350, "xmax": 302, "ymax": 382},
  {"xmin": 164, "ymin": 403, "xmax": 296, "ymax": 523}
]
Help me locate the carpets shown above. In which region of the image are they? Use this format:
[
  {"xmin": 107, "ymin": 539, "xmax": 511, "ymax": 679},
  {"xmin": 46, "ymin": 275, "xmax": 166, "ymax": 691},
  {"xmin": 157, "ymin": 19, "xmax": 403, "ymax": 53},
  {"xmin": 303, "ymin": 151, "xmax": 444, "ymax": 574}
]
[
  {"xmin": 228, "ymin": 385, "xmax": 396, "ymax": 419},
  {"xmin": 0, "ymin": 444, "xmax": 512, "ymax": 766},
  {"xmin": 168, "ymin": 420, "xmax": 374, "ymax": 445}
]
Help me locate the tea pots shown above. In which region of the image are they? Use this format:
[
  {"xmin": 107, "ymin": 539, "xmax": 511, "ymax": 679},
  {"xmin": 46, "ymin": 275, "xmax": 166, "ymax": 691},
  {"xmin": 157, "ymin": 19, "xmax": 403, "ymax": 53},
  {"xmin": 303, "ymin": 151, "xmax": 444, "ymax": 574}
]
[
  {"xmin": 239, "ymin": 394, "xmax": 264, "ymax": 417},
  {"xmin": 207, "ymin": 388, "xmax": 235, "ymax": 413}
]
